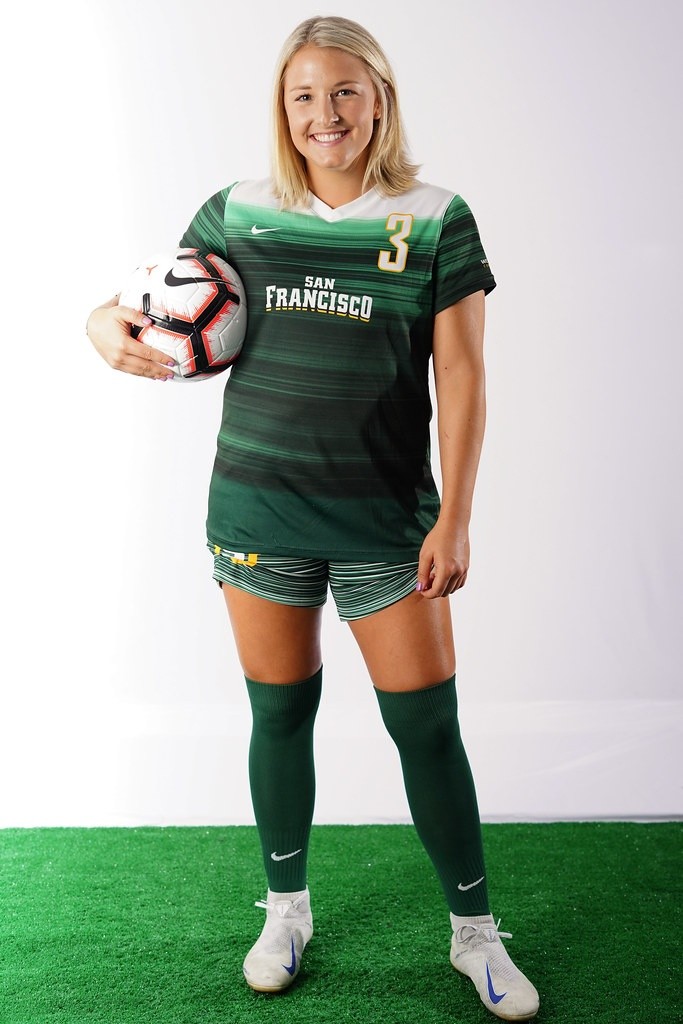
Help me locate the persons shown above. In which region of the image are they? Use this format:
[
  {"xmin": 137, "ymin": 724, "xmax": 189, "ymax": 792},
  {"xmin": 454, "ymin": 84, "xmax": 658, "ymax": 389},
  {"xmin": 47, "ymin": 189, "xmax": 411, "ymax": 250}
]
[{"xmin": 87, "ymin": 18, "xmax": 539, "ymax": 1019}]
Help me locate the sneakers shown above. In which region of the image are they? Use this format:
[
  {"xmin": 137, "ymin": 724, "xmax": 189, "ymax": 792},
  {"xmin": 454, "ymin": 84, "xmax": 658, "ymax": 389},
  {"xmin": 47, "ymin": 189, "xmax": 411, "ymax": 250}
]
[
  {"xmin": 449, "ymin": 919, "xmax": 540, "ymax": 1020},
  {"xmin": 242, "ymin": 891, "xmax": 313, "ymax": 992}
]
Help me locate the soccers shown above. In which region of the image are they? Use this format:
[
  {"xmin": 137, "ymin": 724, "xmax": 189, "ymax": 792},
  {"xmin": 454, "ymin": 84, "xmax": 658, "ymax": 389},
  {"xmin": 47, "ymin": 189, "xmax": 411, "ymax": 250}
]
[{"xmin": 116, "ymin": 245, "xmax": 249, "ymax": 384}]
